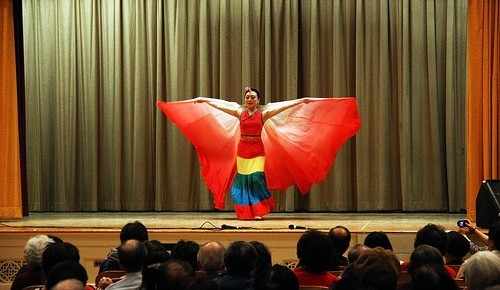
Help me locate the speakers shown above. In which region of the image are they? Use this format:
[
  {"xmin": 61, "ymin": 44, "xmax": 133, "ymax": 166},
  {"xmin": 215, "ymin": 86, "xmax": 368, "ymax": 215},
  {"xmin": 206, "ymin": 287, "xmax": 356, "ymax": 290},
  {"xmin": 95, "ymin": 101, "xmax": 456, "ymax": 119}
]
[{"xmin": 476, "ymin": 179, "xmax": 500, "ymax": 229}]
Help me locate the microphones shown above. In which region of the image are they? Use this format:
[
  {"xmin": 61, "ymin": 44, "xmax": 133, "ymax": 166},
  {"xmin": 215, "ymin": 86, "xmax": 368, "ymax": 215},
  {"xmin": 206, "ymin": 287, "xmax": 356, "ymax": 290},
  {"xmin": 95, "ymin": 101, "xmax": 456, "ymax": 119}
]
[
  {"xmin": 222, "ymin": 224, "xmax": 238, "ymax": 229},
  {"xmin": 289, "ymin": 225, "xmax": 308, "ymax": 229}
]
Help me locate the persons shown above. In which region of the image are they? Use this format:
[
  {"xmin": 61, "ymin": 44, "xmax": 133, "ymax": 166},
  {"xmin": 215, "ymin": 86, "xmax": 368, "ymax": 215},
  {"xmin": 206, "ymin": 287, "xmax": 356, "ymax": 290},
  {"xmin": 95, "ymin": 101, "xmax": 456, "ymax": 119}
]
[
  {"xmin": 11, "ymin": 219, "xmax": 500, "ymax": 290},
  {"xmin": 194, "ymin": 87, "xmax": 310, "ymax": 220}
]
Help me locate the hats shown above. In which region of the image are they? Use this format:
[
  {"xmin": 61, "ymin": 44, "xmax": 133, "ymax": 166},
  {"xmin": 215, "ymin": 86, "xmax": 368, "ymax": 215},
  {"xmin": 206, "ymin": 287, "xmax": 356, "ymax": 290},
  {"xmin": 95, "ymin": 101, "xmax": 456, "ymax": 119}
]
[{"xmin": 353, "ymin": 247, "xmax": 403, "ymax": 284}]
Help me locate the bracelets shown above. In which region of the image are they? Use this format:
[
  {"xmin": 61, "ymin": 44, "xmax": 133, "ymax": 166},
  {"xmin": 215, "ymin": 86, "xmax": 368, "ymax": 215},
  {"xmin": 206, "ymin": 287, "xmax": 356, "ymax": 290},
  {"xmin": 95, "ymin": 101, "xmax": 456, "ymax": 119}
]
[{"xmin": 471, "ymin": 228, "xmax": 477, "ymax": 232}]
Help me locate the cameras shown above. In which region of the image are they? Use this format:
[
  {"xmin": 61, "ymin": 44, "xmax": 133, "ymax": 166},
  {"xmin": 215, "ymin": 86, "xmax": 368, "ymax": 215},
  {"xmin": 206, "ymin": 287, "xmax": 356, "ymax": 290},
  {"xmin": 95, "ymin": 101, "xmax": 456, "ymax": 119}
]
[{"xmin": 457, "ymin": 220, "xmax": 469, "ymax": 227}]
